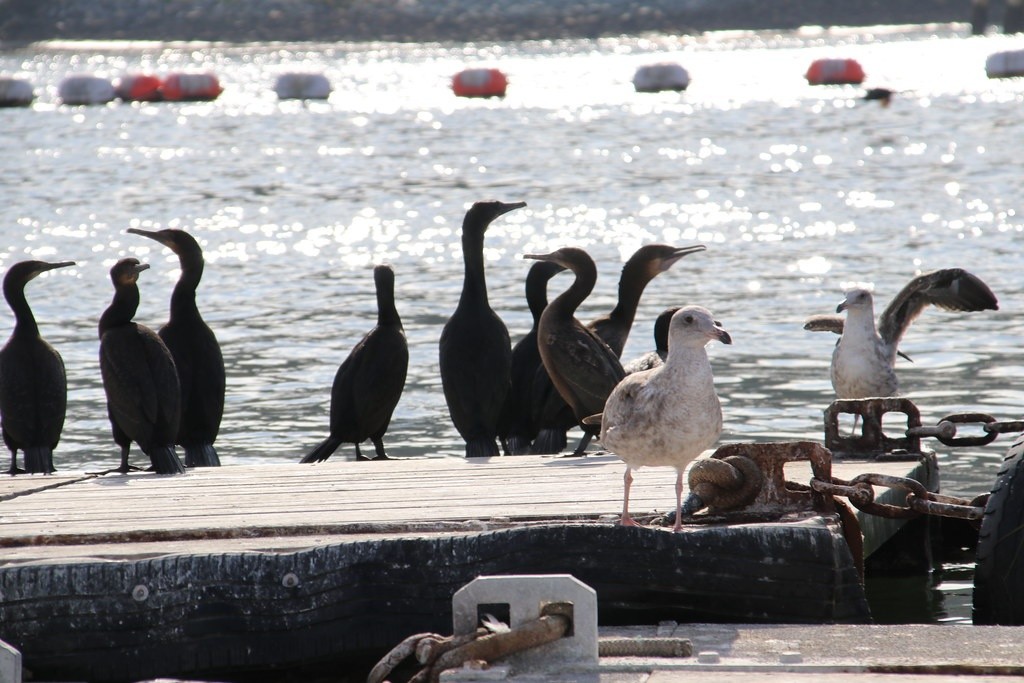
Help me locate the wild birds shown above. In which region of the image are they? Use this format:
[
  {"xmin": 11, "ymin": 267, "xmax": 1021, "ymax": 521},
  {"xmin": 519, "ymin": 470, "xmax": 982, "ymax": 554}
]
[
  {"xmin": 830, "ymin": 286, "xmax": 899, "ymax": 437},
  {"xmin": 1, "ymin": 260, "xmax": 77, "ymax": 471},
  {"xmin": 86, "ymin": 228, "xmax": 225, "ymax": 476},
  {"xmin": 803, "ymin": 268, "xmax": 999, "ymax": 437},
  {"xmin": 439, "ymin": 200, "xmax": 722, "ymax": 456},
  {"xmin": 600, "ymin": 306, "xmax": 732, "ymax": 532},
  {"xmin": 299, "ymin": 265, "xmax": 410, "ymax": 462}
]
